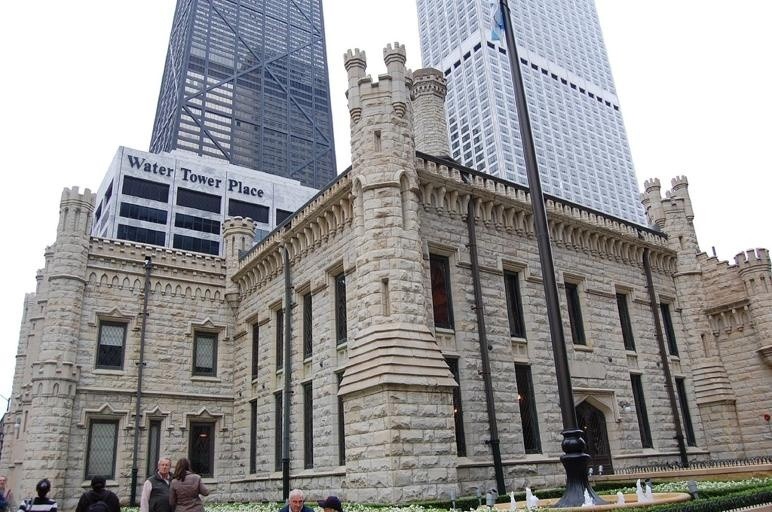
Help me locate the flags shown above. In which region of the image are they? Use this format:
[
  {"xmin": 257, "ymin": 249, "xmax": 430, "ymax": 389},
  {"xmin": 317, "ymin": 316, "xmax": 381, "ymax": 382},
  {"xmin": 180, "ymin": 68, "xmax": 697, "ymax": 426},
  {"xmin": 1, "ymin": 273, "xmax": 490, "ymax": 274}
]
[{"xmin": 489, "ymin": 0, "xmax": 505, "ymax": 42}]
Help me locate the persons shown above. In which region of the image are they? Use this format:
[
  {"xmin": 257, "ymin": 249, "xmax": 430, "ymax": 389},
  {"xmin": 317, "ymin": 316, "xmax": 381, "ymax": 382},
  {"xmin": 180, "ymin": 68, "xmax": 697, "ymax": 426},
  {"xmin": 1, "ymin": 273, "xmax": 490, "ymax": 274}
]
[
  {"xmin": 140, "ymin": 458, "xmax": 177, "ymax": 512},
  {"xmin": 278, "ymin": 488, "xmax": 315, "ymax": 512},
  {"xmin": 168, "ymin": 459, "xmax": 209, "ymax": 512},
  {"xmin": 17, "ymin": 479, "xmax": 57, "ymax": 512},
  {"xmin": 0, "ymin": 476, "xmax": 17, "ymax": 512},
  {"xmin": 75, "ymin": 477, "xmax": 120, "ymax": 512},
  {"xmin": 317, "ymin": 496, "xmax": 343, "ymax": 512}
]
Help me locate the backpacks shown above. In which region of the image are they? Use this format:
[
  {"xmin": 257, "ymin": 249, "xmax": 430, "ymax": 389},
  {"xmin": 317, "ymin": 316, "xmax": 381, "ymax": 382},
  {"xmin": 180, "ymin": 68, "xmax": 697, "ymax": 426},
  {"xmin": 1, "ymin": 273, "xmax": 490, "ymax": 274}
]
[{"xmin": 84, "ymin": 490, "xmax": 111, "ymax": 512}]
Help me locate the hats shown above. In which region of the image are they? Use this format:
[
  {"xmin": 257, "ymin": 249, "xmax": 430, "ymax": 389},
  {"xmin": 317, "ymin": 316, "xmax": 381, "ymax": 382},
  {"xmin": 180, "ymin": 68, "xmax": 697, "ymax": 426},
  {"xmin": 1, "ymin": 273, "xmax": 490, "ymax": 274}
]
[
  {"xmin": 317, "ymin": 496, "xmax": 342, "ymax": 509},
  {"xmin": 36, "ymin": 479, "xmax": 51, "ymax": 491}
]
